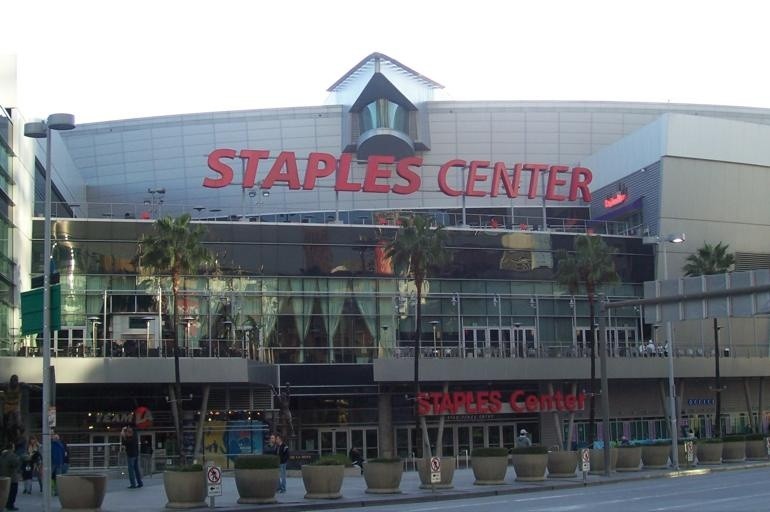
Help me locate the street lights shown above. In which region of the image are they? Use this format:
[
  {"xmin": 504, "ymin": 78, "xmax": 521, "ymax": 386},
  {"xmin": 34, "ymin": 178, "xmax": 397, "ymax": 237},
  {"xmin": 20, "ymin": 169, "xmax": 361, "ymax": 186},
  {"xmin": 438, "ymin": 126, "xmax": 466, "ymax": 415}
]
[
  {"xmin": 528, "ymin": 293, "xmax": 540, "ymax": 357},
  {"xmin": 23, "ymin": 114, "xmax": 76, "ymax": 510},
  {"xmin": 569, "ymin": 296, "xmax": 577, "ymax": 358},
  {"xmin": 634, "ymin": 297, "xmax": 643, "ymax": 358},
  {"xmin": 493, "ymin": 293, "xmax": 502, "ymax": 358},
  {"xmin": 643, "ymin": 233, "xmax": 688, "ymax": 470},
  {"xmin": 603, "ymin": 297, "xmax": 613, "ymax": 357},
  {"xmin": 451, "ymin": 291, "xmax": 463, "ymax": 358}
]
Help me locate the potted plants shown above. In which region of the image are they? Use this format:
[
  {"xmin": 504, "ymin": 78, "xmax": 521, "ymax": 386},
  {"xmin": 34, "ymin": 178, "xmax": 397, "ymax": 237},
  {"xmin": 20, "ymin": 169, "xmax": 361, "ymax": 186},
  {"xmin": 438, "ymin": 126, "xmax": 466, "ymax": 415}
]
[
  {"xmin": 361, "ymin": 456, "xmax": 404, "ymax": 495},
  {"xmin": 468, "ymin": 446, "xmax": 513, "ymax": 485},
  {"xmin": 722, "ymin": 436, "xmax": 747, "ymax": 464},
  {"xmin": 695, "ymin": 437, "xmax": 725, "ymax": 466},
  {"xmin": 300, "ymin": 452, "xmax": 348, "ymax": 500},
  {"xmin": 232, "ymin": 454, "xmax": 283, "ymax": 505},
  {"xmin": 668, "ymin": 436, "xmax": 700, "ymax": 470},
  {"xmin": 746, "ymin": 435, "xmax": 768, "ymax": 462},
  {"xmin": 642, "ymin": 438, "xmax": 672, "ymax": 470},
  {"xmin": 163, "ymin": 462, "xmax": 209, "ymax": 510},
  {"xmin": 614, "ymin": 440, "xmax": 644, "ymax": 472},
  {"xmin": 509, "ymin": 445, "xmax": 549, "ymax": 482}
]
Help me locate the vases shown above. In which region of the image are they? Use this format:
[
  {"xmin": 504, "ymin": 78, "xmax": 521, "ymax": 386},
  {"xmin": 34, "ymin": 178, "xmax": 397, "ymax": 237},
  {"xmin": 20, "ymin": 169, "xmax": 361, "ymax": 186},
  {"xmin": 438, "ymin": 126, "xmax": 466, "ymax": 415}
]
[
  {"xmin": 55, "ymin": 473, "xmax": 106, "ymax": 512},
  {"xmin": 0, "ymin": 477, "xmax": 12, "ymax": 512}
]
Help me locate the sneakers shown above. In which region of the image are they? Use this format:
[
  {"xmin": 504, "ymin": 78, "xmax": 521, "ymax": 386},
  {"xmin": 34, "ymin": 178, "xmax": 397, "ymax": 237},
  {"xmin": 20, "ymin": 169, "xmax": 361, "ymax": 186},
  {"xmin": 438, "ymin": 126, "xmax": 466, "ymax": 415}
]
[
  {"xmin": 275, "ymin": 486, "xmax": 287, "ymax": 494},
  {"xmin": 127, "ymin": 483, "xmax": 143, "ymax": 488},
  {"xmin": 6, "ymin": 489, "xmax": 60, "ymax": 511}
]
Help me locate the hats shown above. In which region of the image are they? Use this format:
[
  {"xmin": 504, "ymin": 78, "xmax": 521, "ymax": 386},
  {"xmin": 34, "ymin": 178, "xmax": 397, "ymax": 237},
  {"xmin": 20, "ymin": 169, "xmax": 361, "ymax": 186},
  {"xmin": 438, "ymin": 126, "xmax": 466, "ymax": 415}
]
[{"xmin": 520, "ymin": 429, "xmax": 528, "ymax": 434}]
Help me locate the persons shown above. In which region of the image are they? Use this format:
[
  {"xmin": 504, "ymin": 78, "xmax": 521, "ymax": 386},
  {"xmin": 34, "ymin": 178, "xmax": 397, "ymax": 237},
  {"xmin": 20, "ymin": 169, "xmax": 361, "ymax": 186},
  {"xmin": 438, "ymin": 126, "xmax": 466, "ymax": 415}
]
[
  {"xmin": 276, "ymin": 435, "xmax": 289, "ymax": 493},
  {"xmin": 263, "ymin": 434, "xmax": 278, "ymax": 453},
  {"xmin": 269, "ymin": 382, "xmax": 295, "ymax": 437},
  {"xmin": 516, "ymin": 428, "xmax": 532, "ymax": 448},
  {"xmin": 0, "ymin": 376, "xmax": 42, "ymax": 439},
  {"xmin": 350, "ymin": 447, "xmax": 363, "ymax": 474},
  {"xmin": 640, "ymin": 340, "xmax": 669, "ymax": 358},
  {"xmin": 0, "ymin": 434, "xmax": 69, "ymax": 512},
  {"xmin": 120, "ymin": 426, "xmax": 143, "ymax": 488}
]
